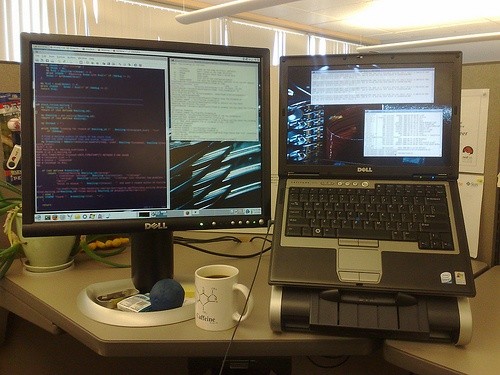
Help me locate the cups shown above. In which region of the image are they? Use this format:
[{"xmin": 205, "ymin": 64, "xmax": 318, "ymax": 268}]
[{"xmin": 194, "ymin": 265, "xmax": 254, "ymax": 332}]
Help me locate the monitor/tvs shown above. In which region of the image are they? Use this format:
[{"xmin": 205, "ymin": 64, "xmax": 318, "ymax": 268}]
[{"xmin": 20, "ymin": 32, "xmax": 272, "ymax": 238}]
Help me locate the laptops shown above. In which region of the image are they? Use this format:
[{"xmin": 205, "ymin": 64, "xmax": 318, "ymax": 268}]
[{"xmin": 267, "ymin": 51, "xmax": 476, "ymax": 294}]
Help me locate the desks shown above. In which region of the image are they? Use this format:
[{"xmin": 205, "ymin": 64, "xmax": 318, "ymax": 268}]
[{"xmin": 1, "ymin": 233, "xmax": 500, "ymax": 375}]
